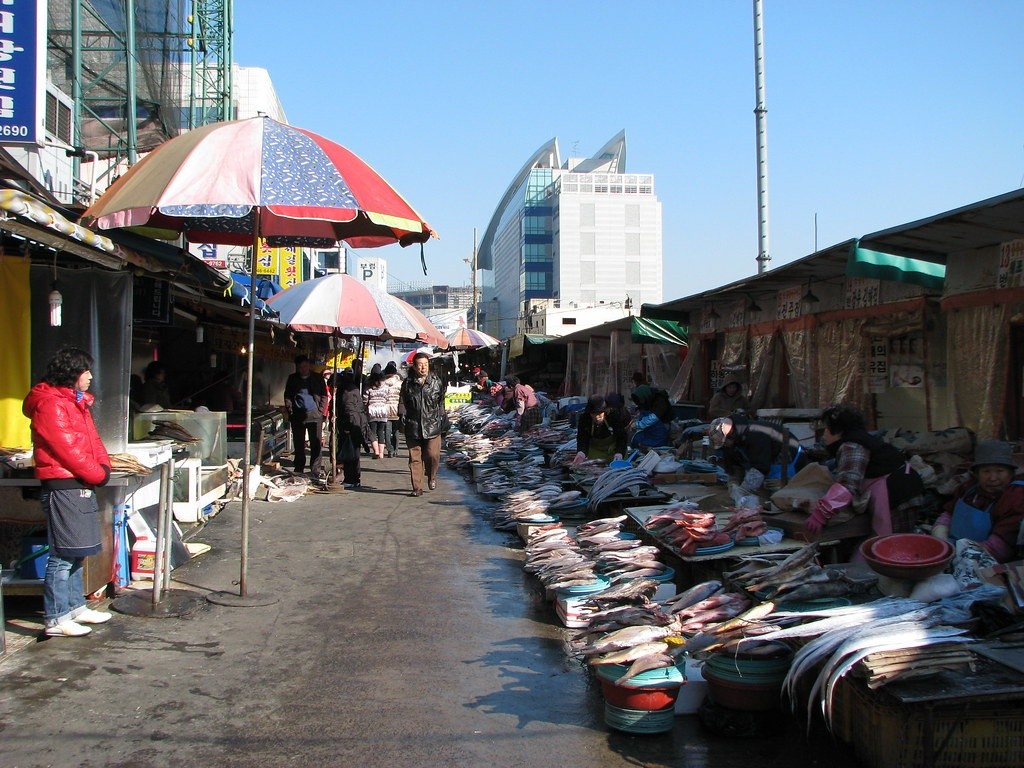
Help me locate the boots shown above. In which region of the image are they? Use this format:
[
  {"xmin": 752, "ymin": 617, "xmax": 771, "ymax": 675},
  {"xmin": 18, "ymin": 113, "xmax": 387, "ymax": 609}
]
[
  {"xmin": 379, "ymin": 444, "xmax": 384, "ymax": 458},
  {"xmin": 372, "ymin": 441, "xmax": 379, "ymax": 459},
  {"xmin": 332, "ymin": 464, "xmax": 344, "ymax": 482}
]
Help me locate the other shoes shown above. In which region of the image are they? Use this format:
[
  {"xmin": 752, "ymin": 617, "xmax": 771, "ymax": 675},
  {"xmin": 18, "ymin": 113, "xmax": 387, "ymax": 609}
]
[
  {"xmin": 393, "ymin": 452, "xmax": 399, "ymax": 457},
  {"xmin": 410, "ymin": 489, "xmax": 423, "ymax": 497},
  {"xmin": 428, "ymin": 478, "xmax": 436, "ymax": 490},
  {"xmin": 388, "ymin": 453, "xmax": 393, "ymax": 458},
  {"xmin": 341, "ymin": 478, "xmax": 361, "ymax": 490}
]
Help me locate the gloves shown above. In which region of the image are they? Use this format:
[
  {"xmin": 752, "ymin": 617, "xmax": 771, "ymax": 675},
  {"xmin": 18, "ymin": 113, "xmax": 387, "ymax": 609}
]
[
  {"xmin": 516, "ymin": 415, "xmax": 522, "ymax": 429},
  {"xmin": 805, "ymin": 510, "xmax": 828, "ymax": 535},
  {"xmin": 574, "ymin": 451, "xmax": 585, "ymax": 464},
  {"xmin": 614, "ymin": 453, "xmax": 622, "ymax": 460},
  {"xmin": 399, "ymin": 415, "xmax": 405, "ymax": 428}
]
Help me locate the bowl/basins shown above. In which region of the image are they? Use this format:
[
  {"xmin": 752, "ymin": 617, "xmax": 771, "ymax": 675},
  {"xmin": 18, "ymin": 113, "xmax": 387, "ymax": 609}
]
[
  {"xmin": 860, "ymin": 532, "xmax": 955, "ymax": 580},
  {"xmin": 701, "ymin": 653, "xmax": 789, "ymax": 709},
  {"xmin": 595, "ymin": 658, "xmax": 689, "ymax": 734}
]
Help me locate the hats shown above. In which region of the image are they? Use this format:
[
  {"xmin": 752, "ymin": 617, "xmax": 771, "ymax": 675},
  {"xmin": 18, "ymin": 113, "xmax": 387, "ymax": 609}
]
[
  {"xmin": 473, "ymin": 368, "xmax": 480, "ymax": 373},
  {"xmin": 371, "ymin": 363, "xmax": 382, "ymax": 374},
  {"xmin": 969, "ymin": 439, "xmax": 1019, "ymax": 472},
  {"xmin": 585, "ymin": 394, "xmax": 607, "ymax": 413},
  {"xmin": 710, "ymin": 417, "xmax": 733, "ymax": 449},
  {"xmin": 323, "ymin": 370, "xmax": 332, "ymax": 376},
  {"xmin": 341, "ymin": 367, "xmax": 354, "ymax": 374}
]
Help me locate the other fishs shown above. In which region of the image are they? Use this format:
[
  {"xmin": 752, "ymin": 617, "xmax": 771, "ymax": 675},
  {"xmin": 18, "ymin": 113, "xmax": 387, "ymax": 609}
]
[
  {"xmin": 445, "ymin": 399, "xmax": 983, "ymax": 735},
  {"xmin": 103, "ymin": 453, "xmax": 153, "ymax": 475},
  {"xmin": 147, "ymin": 420, "xmax": 202, "ymax": 442}
]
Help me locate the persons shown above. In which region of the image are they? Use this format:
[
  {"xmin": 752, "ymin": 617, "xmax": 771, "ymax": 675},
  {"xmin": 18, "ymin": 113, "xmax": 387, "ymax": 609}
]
[
  {"xmin": 930, "ymin": 439, "xmax": 1024, "ymax": 564},
  {"xmin": 625, "ymin": 384, "xmax": 668, "ymax": 463},
  {"xmin": 24, "ymin": 349, "xmax": 113, "ymax": 638},
  {"xmin": 326, "ymin": 359, "xmax": 405, "ymax": 488},
  {"xmin": 284, "ymin": 354, "xmax": 328, "ymax": 477},
  {"xmin": 803, "ymin": 405, "xmax": 928, "ymax": 537},
  {"xmin": 573, "ymin": 394, "xmax": 627, "ymax": 465},
  {"xmin": 708, "ymin": 417, "xmax": 808, "ymax": 502},
  {"xmin": 469, "ymin": 367, "xmax": 516, "ymax": 416},
  {"xmin": 708, "ymin": 374, "xmax": 749, "ymax": 422},
  {"xmin": 506, "ymin": 376, "xmax": 539, "ymax": 437},
  {"xmin": 397, "ymin": 353, "xmax": 450, "ymax": 497},
  {"xmin": 629, "ymin": 371, "xmax": 643, "ymax": 401},
  {"xmin": 142, "ymin": 360, "xmax": 171, "ymax": 409}
]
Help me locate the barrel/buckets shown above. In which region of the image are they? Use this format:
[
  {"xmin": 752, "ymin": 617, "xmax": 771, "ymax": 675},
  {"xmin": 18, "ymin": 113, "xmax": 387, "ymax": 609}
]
[{"xmin": 130, "ymin": 536, "xmax": 166, "ymax": 581}]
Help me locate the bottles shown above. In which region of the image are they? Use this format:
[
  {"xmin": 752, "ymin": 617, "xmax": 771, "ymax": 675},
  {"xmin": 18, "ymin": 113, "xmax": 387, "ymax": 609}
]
[{"xmin": 702, "ymin": 436, "xmax": 709, "ymax": 445}]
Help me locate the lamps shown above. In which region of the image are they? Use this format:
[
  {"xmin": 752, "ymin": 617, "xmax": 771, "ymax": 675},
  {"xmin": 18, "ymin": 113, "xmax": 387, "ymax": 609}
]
[
  {"xmin": 706, "ymin": 299, "xmax": 720, "ymax": 328},
  {"xmin": 745, "ymin": 289, "xmax": 774, "ymax": 319},
  {"xmin": 802, "ymin": 274, "xmax": 843, "ymax": 308}
]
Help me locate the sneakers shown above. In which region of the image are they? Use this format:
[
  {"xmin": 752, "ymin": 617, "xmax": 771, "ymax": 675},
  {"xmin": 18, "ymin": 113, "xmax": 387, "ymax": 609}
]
[
  {"xmin": 45, "ymin": 620, "xmax": 92, "ymax": 636},
  {"xmin": 72, "ymin": 609, "xmax": 112, "ymax": 623}
]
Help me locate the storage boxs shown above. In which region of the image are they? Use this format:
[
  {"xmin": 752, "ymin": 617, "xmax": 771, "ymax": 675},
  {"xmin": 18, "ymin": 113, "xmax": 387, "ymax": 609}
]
[
  {"xmin": 22, "ymin": 540, "xmax": 50, "ymax": 579},
  {"xmin": 610, "ymin": 508, "xmax": 1024, "ymax": 768},
  {"xmin": 785, "ymin": 423, "xmax": 815, "ymax": 449},
  {"xmin": 516, "ymin": 523, "xmax": 676, "ymax": 627},
  {"xmin": 132, "ymin": 411, "xmax": 227, "ymax": 508}
]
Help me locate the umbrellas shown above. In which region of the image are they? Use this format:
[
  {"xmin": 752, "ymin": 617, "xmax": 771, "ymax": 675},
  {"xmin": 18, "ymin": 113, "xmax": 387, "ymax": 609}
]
[
  {"xmin": 245, "ymin": 273, "xmax": 449, "ymax": 483},
  {"xmin": 399, "ymin": 348, "xmax": 434, "ymax": 363},
  {"xmin": 452, "ymin": 351, "xmax": 462, "ymax": 388},
  {"xmin": 432, "ymin": 327, "xmax": 502, "ymax": 389},
  {"xmin": 76, "ymin": 115, "xmax": 441, "ymax": 598}
]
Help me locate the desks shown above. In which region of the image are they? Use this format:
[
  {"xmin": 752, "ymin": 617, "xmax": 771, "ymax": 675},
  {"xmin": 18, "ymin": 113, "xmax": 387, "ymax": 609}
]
[
  {"xmin": 625, "ymin": 504, "xmax": 812, "ymax": 561},
  {"xmin": 652, "ymin": 473, "xmax": 717, "ymax": 483},
  {"xmin": 0, "ymin": 452, "xmax": 189, "ymax": 604},
  {"xmin": 570, "ymin": 474, "xmax": 667, "ymax": 502}
]
